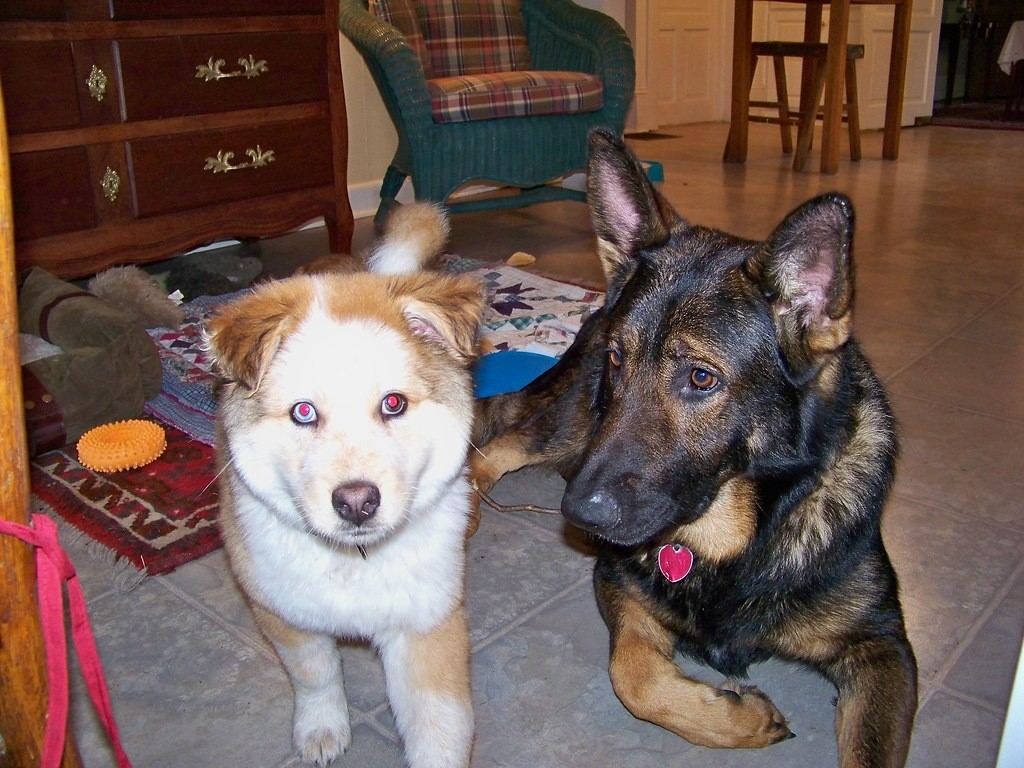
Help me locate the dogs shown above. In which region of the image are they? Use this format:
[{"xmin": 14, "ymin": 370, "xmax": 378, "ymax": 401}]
[
  {"xmin": 462, "ymin": 125, "xmax": 919, "ymax": 768},
  {"xmin": 196, "ymin": 200, "xmax": 490, "ymax": 768}
]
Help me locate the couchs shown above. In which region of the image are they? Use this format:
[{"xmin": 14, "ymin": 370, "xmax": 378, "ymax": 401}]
[{"xmin": 336, "ymin": 1, "xmax": 636, "ymax": 237}]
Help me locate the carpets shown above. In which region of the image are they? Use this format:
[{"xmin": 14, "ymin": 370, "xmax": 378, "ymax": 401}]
[{"xmin": 29, "ymin": 277, "xmax": 605, "ymax": 597}]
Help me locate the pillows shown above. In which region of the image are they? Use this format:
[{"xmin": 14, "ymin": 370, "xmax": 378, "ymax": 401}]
[{"xmin": 19, "ymin": 264, "xmax": 162, "ymax": 399}]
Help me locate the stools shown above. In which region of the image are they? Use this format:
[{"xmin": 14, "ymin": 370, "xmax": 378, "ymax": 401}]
[{"xmin": 715, "ymin": 39, "xmax": 865, "ymax": 170}]
